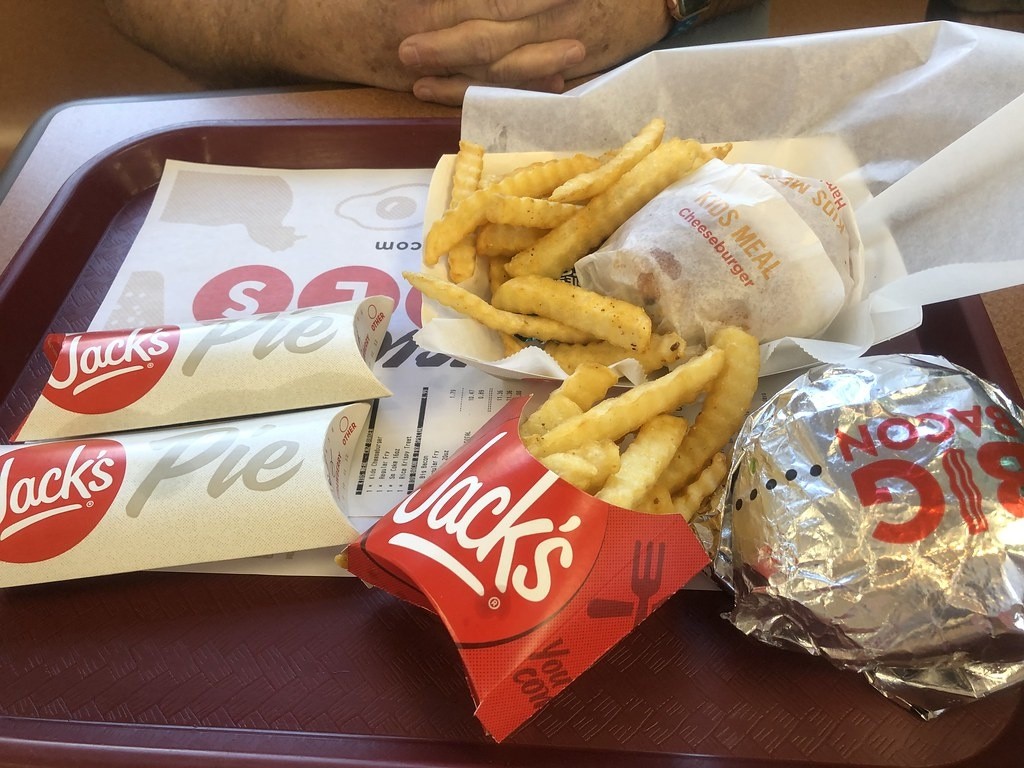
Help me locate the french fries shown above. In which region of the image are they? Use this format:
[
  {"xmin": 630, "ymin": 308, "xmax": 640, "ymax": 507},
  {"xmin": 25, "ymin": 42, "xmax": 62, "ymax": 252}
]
[{"xmin": 401, "ymin": 118, "xmax": 762, "ymax": 526}]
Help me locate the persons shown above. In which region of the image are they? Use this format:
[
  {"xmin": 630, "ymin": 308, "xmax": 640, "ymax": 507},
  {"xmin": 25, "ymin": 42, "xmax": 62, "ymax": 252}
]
[{"xmin": 105, "ymin": 0, "xmax": 770, "ymax": 107}]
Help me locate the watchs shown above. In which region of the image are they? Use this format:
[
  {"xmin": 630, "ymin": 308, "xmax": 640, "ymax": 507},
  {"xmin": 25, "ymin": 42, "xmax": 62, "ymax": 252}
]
[{"xmin": 660, "ymin": 0, "xmax": 710, "ymax": 41}]
